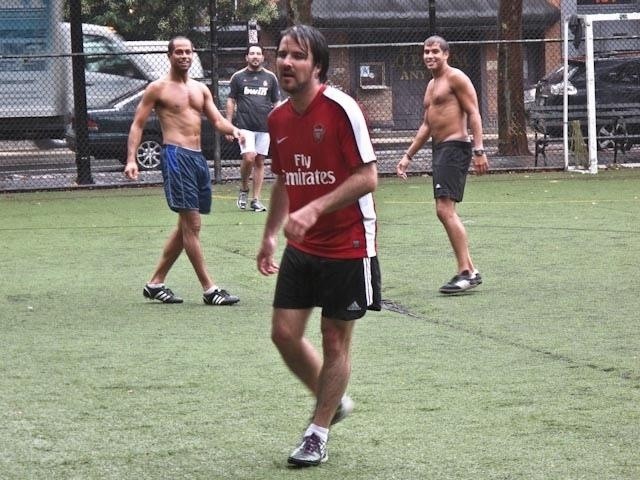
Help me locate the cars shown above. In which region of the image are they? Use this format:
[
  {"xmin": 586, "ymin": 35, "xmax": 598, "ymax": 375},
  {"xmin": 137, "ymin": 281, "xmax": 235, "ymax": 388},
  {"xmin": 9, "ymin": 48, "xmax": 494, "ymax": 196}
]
[{"xmin": 63, "ymin": 78, "xmax": 243, "ymax": 174}]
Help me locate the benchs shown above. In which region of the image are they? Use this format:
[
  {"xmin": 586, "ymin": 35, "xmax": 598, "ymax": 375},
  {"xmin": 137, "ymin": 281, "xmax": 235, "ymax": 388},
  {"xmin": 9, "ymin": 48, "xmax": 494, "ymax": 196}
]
[{"xmin": 528, "ymin": 99, "xmax": 640, "ymax": 168}]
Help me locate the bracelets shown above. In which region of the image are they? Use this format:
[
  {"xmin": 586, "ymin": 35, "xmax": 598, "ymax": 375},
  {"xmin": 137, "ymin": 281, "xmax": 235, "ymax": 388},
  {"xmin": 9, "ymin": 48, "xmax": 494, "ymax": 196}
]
[{"xmin": 404, "ymin": 149, "xmax": 412, "ymax": 162}]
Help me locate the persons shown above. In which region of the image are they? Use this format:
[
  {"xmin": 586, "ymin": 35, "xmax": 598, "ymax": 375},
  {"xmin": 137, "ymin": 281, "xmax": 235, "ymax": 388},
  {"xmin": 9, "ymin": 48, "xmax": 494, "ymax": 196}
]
[
  {"xmin": 224, "ymin": 43, "xmax": 280, "ymax": 213},
  {"xmin": 394, "ymin": 34, "xmax": 489, "ymax": 295},
  {"xmin": 124, "ymin": 35, "xmax": 246, "ymax": 306},
  {"xmin": 256, "ymin": 24, "xmax": 383, "ymax": 463}
]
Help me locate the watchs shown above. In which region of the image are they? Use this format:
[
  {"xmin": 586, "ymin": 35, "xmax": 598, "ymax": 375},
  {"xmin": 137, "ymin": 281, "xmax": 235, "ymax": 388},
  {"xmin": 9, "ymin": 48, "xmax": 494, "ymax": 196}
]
[{"xmin": 473, "ymin": 147, "xmax": 486, "ymax": 156}]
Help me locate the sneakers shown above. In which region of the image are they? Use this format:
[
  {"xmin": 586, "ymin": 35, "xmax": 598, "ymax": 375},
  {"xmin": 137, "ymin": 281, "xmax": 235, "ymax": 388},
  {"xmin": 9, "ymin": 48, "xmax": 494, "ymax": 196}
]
[
  {"xmin": 438, "ymin": 268, "xmax": 485, "ymax": 295},
  {"xmin": 140, "ymin": 282, "xmax": 185, "ymax": 306},
  {"xmin": 285, "ymin": 433, "xmax": 331, "ymax": 467},
  {"xmin": 299, "ymin": 392, "xmax": 357, "ymax": 433},
  {"xmin": 200, "ymin": 287, "xmax": 242, "ymax": 307},
  {"xmin": 249, "ymin": 196, "xmax": 269, "ymax": 214},
  {"xmin": 235, "ymin": 185, "xmax": 251, "ymax": 211}
]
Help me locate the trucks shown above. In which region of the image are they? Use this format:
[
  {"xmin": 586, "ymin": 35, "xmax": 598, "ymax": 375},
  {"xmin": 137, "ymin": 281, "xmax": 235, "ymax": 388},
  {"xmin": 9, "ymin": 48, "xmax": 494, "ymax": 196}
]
[
  {"xmin": 0, "ymin": 0, "xmax": 171, "ymax": 143},
  {"xmin": 77, "ymin": 34, "xmax": 207, "ymax": 82}
]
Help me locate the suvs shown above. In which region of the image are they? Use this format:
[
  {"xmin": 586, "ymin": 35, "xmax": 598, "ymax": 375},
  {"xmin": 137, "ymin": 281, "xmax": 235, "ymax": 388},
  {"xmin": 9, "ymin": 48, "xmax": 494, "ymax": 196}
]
[
  {"xmin": 522, "ymin": 54, "xmax": 588, "ymax": 137},
  {"xmin": 526, "ymin": 53, "xmax": 640, "ymax": 153}
]
